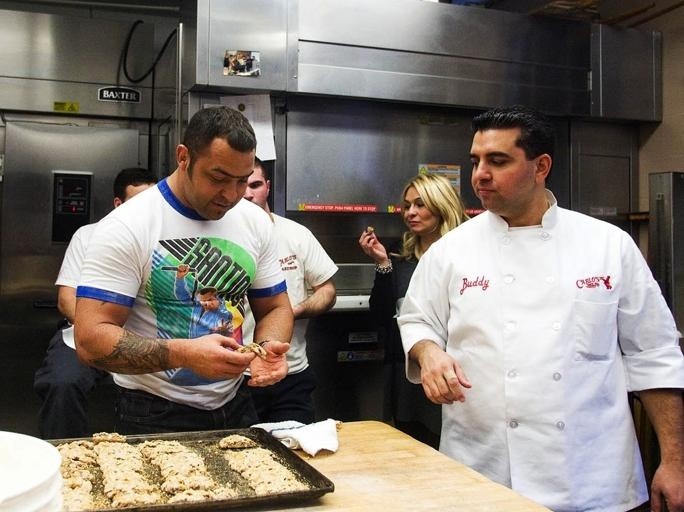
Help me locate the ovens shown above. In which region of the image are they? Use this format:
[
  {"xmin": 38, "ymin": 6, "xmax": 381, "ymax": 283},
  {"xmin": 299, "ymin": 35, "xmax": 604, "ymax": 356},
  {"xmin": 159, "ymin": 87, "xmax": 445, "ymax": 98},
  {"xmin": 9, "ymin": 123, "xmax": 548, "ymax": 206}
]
[{"xmin": 1, "ymin": 108, "xmax": 151, "ymax": 440}]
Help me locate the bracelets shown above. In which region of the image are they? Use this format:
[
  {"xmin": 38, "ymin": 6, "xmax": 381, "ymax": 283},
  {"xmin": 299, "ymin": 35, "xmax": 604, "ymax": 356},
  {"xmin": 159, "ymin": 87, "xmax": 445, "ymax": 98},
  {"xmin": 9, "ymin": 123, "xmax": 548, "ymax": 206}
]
[
  {"xmin": 373, "ymin": 259, "xmax": 392, "ymax": 273},
  {"xmin": 257, "ymin": 340, "xmax": 268, "ymax": 353}
]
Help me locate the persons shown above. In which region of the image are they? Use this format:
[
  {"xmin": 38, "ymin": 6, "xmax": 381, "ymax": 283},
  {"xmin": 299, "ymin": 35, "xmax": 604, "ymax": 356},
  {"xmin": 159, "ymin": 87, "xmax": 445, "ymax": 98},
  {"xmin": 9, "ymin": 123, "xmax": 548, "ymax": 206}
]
[
  {"xmin": 357, "ymin": 172, "xmax": 470, "ymax": 450},
  {"xmin": 73, "ymin": 106, "xmax": 294, "ymax": 433},
  {"xmin": 242, "ymin": 153, "xmax": 339, "ymax": 424},
  {"xmin": 32, "ymin": 167, "xmax": 158, "ymax": 441},
  {"xmin": 395, "ymin": 103, "xmax": 684, "ymax": 510}
]
